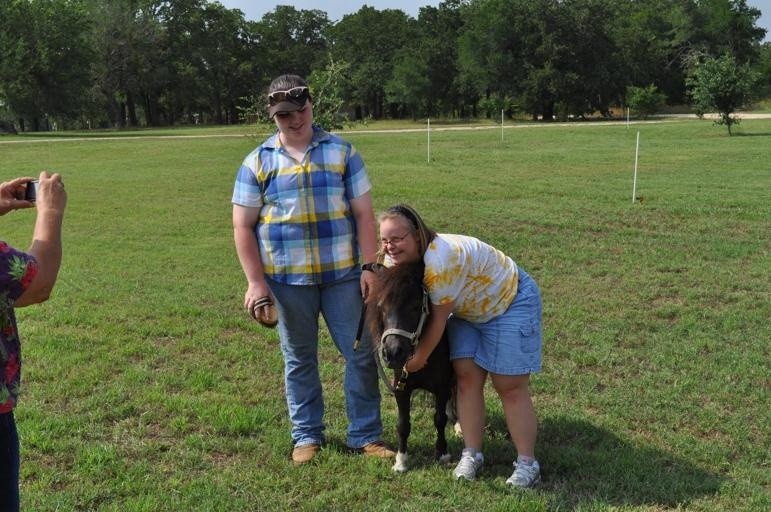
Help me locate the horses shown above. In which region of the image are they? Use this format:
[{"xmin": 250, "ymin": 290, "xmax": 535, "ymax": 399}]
[{"xmin": 362, "ymin": 258, "xmax": 466, "ymax": 476}]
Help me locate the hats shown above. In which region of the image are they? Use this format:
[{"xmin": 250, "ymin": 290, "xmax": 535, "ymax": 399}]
[{"xmin": 269, "ymin": 96, "xmax": 309, "ymax": 119}]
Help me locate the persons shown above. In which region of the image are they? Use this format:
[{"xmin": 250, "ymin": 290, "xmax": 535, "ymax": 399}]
[
  {"xmin": 0, "ymin": 170, "xmax": 68, "ymax": 512},
  {"xmin": 230, "ymin": 74, "xmax": 397, "ymax": 466},
  {"xmin": 378, "ymin": 204, "xmax": 542, "ymax": 489}
]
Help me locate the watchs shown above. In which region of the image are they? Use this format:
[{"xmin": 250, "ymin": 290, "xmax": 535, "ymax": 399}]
[{"xmin": 362, "ymin": 263, "xmax": 377, "ymax": 272}]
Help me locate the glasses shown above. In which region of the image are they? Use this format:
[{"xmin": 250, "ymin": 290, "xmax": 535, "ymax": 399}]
[
  {"xmin": 268, "ymin": 86, "xmax": 309, "ymax": 103},
  {"xmin": 380, "ymin": 228, "xmax": 414, "ymax": 248}
]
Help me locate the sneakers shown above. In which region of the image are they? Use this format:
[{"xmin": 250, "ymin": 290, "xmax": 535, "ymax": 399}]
[
  {"xmin": 505, "ymin": 454, "xmax": 542, "ymax": 490},
  {"xmin": 292, "ymin": 444, "xmax": 320, "ymax": 464},
  {"xmin": 452, "ymin": 448, "xmax": 485, "ymax": 483},
  {"xmin": 351, "ymin": 440, "xmax": 397, "ymax": 459}
]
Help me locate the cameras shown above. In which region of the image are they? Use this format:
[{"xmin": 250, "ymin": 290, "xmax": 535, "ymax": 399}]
[{"xmin": 24, "ymin": 181, "xmax": 38, "ymax": 202}]
